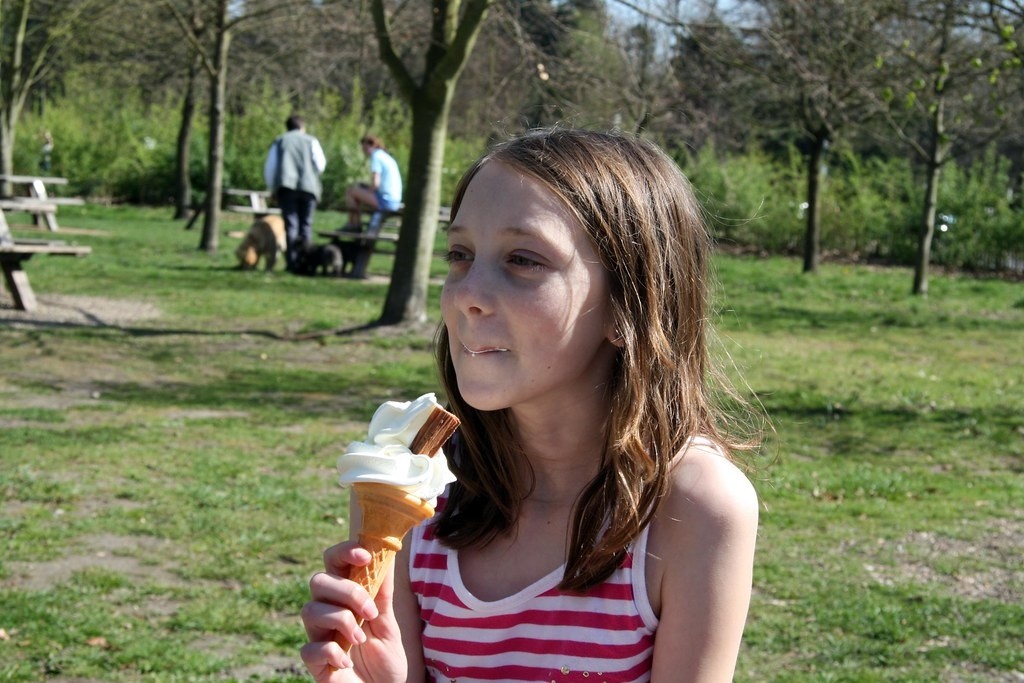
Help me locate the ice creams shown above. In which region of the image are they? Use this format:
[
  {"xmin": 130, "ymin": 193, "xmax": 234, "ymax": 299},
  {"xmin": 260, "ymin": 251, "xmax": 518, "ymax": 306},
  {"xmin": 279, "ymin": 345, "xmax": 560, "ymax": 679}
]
[{"xmin": 334, "ymin": 391, "xmax": 460, "ymax": 655}]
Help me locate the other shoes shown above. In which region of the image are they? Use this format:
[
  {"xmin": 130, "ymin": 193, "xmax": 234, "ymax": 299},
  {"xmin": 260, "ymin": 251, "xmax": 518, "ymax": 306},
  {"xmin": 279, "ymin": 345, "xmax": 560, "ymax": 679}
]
[
  {"xmin": 343, "ymin": 271, "xmax": 364, "ymax": 281},
  {"xmin": 337, "ymin": 223, "xmax": 363, "ymax": 234}
]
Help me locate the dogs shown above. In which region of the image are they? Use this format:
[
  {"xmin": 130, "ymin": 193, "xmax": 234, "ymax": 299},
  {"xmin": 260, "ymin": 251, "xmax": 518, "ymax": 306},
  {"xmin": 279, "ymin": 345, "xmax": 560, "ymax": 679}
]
[{"xmin": 234, "ymin": 214, "xmax": 342, "ymax": 280}]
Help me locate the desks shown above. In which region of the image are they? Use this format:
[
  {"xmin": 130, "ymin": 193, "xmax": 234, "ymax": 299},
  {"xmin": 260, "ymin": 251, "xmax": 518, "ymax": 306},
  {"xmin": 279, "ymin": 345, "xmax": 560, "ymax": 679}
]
[{"xmin": 0, "ymin": 176, "xmax": 69, "ymax": 233}]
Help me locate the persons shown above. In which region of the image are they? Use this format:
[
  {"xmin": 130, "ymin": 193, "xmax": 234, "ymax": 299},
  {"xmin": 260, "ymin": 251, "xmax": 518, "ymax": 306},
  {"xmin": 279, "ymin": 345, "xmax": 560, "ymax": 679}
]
[
  {"xmin": 301, "ymin": 124, "xmax": 759, "ymax": 683},
  {"xmin": 339, "ymin": 136, "xmax": 402, "ymax": 233},
  {"xmin": 264, "ymin": 115, "xmax": 325, "ymax": 271}
]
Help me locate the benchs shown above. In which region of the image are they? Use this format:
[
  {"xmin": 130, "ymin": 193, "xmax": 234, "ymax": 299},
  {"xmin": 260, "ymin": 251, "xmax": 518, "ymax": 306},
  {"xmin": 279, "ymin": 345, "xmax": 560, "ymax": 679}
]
[
  {"xmin": 226, "ymin": 189, "xmax": 407, "ymax": 280},
  {"xmin": 0, "ymin": 176, "xmax": 105, "ymax": 326}
]
